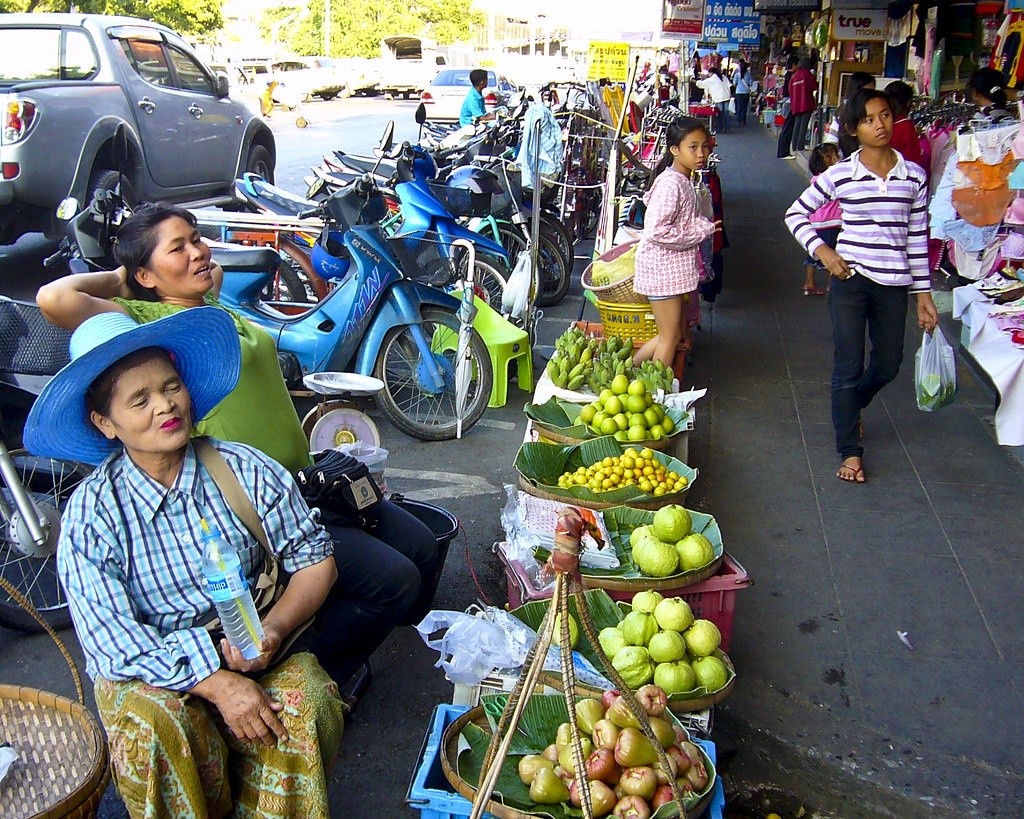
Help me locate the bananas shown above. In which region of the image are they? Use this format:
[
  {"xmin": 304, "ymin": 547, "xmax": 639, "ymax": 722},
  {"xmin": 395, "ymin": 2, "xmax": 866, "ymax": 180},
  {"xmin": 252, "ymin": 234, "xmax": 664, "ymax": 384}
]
[{"xmin": 546, "ymin": 326, "xmax": 674, "ymax": 396}]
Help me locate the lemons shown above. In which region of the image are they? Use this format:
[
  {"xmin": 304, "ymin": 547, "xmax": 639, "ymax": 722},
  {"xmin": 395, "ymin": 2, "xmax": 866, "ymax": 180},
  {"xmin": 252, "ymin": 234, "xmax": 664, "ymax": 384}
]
[{"xmin": 557, "ymin": 447, "xmax": 689, "ymax": 497}]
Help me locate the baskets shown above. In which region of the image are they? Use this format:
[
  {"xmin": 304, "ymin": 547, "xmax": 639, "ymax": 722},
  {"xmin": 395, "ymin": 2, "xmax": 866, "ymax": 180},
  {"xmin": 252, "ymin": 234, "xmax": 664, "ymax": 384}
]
[
  {"xmin": 534, "ymin": 647, "xmax": 736, "ymax": 712},
  {"xmin": 581, "ymin": 240, "xmax": 658, "ymax": 341},
  {"xmin": 578, "ymin": 509, "xmax": 724, "ymax": 591},
  {"xmin": 531, "ymin": 418, "xmax": 682, "ymax": 452},
  {"xmin": 517, "ymin": 473, "xmax": 689, "ymax": 509},
  {"xmin": 440, "ymin": 507, "xmax": 718, "ymax": 819},
  {"xmin": 0, "ymin": 574, "xmax": 112, "ymax": 819}
]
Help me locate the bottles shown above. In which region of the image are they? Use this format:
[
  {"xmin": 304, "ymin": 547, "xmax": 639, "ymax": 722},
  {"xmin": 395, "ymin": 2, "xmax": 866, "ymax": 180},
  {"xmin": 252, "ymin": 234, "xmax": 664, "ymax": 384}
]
[{"xmin": 200, "ymin": 518, "xmax": 267, "ymax": 660}]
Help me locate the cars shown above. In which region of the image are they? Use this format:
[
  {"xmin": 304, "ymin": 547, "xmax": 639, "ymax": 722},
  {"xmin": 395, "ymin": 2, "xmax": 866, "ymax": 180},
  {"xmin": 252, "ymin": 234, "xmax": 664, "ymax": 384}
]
[
  {"xmin": 420, "ymin": 66, "xmax": 520, "ymax": 127},
  {"xmin": 0, "ymin": 13, "xmax": 277, "ymax": 261},
  {"xmin": 199, "ymin": 35, "xmax": 450, "ymax": 107}
]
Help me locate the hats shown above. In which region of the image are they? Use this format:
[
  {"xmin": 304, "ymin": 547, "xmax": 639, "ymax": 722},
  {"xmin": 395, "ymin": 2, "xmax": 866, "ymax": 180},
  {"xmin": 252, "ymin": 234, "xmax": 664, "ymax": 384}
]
[{"xmin": 22, "ymin": 306, "xmax": 242, "ymax": 466}]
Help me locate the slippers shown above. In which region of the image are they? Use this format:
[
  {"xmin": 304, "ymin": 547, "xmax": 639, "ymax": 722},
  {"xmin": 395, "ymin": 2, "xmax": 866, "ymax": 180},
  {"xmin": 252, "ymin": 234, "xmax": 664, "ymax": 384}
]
[{"xmin": 839, "ymin": 462, "xmax": 866, "ymax": 483}]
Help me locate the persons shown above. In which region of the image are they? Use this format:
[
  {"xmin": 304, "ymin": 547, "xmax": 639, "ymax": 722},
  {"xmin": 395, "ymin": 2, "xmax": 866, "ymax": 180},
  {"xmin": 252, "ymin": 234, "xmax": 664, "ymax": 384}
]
[
  {"xmin": 54, "ymin": 312, "xmax": 340, "ymax": 819},
  {"xmin": 776, "ymin": 55, "xmax": 803, "ymax": 160},
  {"xmin": 691, "ymin": 57, "xmax": 754, "ymax": 133},
  {"xmin": 788, "ymin": 58, "xmax": 818, "ymax": 151},
  {"xmin": 38, "ymin": 205, "xmax": 441, "ymax": 708},
  {"xmin": 801, "ymin": 71, "xmax": 922, "ymax": 297},
  {"xmin": 459, "ymin": 70, "xmax": 488, "ymax": 126},
  {"xmin": 782, "ymin": 90, "xmax": 938, "ymax": 485},
  {"xmin": 968, "ymin": 67, "xmax": 1008, "ymax": 109},
  {"xmin": 632, "ymin": 117, "xmax": 723, "ymax": 373}
]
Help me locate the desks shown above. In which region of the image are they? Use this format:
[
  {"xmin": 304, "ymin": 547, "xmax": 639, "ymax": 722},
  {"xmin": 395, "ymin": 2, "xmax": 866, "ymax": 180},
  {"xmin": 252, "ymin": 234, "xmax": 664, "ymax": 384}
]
[
  {"xmin": 688, "ymin": 102, "xmax": 719, "ymax": 136},
  {"xmin": 952, "ymin": 268, "xmax": 1024, "ymax": 447}
]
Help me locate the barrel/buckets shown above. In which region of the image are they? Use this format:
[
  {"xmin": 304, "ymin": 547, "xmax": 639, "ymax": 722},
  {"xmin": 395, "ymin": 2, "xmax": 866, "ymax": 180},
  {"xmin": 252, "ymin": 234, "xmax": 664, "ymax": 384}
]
[{"xmin": 386, "ymin": 492, "xmax": 460, "ymax": 626}]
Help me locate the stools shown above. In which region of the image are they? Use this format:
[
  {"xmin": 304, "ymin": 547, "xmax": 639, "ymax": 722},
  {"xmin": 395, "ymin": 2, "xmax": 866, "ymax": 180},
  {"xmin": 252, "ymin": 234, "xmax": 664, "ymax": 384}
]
[{"xmin": 423, "ymin": 288, "xmax": 534, "ymax": 409}]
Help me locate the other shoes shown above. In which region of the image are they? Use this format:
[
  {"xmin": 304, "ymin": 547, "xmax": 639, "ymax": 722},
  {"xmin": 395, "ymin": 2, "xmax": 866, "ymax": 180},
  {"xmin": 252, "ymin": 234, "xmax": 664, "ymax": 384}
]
[
  {"xmin": 779, "ymin": 155, "xmax": 795, "ymax": 159},
  {"xmin": 802, "ymin": 284, "xmax": 823, "ymax": 295}
]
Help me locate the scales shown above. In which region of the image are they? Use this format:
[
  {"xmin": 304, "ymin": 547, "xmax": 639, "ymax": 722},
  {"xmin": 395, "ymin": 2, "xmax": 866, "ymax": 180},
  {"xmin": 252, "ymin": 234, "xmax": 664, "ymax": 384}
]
[{"xmin": 299, "ymin": 372, "xmax": 385, "ymax": 452}]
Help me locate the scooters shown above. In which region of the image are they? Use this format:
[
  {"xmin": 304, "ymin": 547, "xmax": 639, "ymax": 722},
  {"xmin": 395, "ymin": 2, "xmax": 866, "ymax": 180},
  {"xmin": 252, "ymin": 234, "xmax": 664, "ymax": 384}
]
[{"xmin": 0, "ymin": 82, "xmax": 621, "ymax": 633}]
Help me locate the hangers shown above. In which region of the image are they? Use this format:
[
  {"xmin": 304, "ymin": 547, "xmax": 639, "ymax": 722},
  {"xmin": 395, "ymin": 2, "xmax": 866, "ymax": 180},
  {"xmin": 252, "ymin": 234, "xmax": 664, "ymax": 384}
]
[{"xmin": 914, "ymin": 89, "xmax": 981, "ymax": 131}]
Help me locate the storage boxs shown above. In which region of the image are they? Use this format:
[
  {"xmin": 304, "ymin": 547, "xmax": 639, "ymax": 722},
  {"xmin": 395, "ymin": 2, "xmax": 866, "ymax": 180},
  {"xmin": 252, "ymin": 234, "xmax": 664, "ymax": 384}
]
[
  {"xmin": 572, "ymin": 322, "xmax": 686, "ymax": 382},
  {"xmin": 492, "ymin": 540, "xmax": 754, "ymax": 653},
  {"xmin": 403, "ymin": 703, "xmax": 726, "ymax": 819},
  {"xmin": 450, "ymin": 663, "xmax": 716, "ymax": 740}
]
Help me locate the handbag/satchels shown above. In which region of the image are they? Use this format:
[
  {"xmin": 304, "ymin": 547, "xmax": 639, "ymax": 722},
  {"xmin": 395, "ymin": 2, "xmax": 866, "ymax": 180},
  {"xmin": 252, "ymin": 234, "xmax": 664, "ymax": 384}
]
[
  {"xmin": 296, "ymin": 447, "xmax": 384, "ymax": 530},
  {"xmin": 914, "ymin": 322, "xmax": 957, "ymax": 412}
]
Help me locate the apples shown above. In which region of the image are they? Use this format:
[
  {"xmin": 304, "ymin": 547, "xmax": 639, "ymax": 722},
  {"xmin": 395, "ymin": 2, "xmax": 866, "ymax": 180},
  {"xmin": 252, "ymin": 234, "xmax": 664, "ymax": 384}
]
[{"xmin": 572, "ymin": 373, "xmax": 674, "ymax": 441}]
